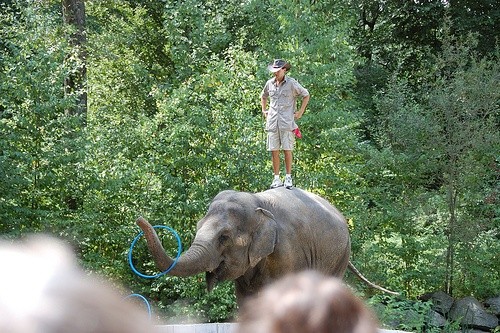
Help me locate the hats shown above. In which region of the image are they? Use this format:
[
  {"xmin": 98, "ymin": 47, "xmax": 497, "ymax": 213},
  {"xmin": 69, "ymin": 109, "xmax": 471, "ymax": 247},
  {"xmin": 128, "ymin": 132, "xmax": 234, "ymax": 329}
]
[{"xmin": 268, "ymin": 59, "xmax": 291, "ymax": 72}]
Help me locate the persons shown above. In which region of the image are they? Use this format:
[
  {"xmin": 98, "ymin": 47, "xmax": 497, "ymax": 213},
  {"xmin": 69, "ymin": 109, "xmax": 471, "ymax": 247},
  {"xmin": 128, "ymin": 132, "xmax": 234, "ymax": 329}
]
[
  {"xmin": 260, "ymin": 59, "xmax": 310, "ymax": 189},
  {"xmin": 234, "ymin": 268, "xmax": 379, "ymax": 333}
]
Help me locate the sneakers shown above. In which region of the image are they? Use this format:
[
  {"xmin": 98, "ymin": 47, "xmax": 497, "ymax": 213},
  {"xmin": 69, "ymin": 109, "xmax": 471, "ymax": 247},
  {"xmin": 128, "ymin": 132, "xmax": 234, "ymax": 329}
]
[
  {"xmin": 270, "ymin": 178, "xmax": 283, "ymax": 189},
  {"xmin": 284, "ymin": 176, "xmax": 292, "ymax": 187}
]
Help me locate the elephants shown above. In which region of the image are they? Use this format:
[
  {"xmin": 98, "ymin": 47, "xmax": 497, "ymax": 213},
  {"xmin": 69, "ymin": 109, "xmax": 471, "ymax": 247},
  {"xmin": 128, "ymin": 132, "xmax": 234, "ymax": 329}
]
[{"xmin": 135, "ymin": 183, "xmax": 400, "ymax": 312}]
